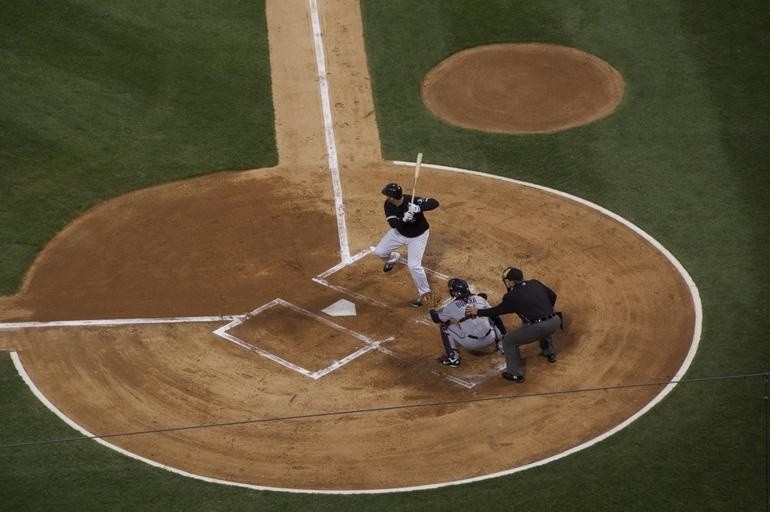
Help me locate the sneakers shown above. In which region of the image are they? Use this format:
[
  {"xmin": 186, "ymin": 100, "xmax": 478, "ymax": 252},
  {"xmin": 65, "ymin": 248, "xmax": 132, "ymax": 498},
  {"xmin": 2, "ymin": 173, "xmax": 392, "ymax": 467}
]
[
  {"xmin": 502, "ymin": 372, "xmax": 525, "ymax": 383},
  {"xmin": 409, "ymin": 297, "xmax": 424, "ymax": 307},
  {"xmin": 442, "ymin": 353, "xmax": 461, "ymax": 368},
  {"xmin": 383, "ymin": 262, "xmax": 393, "ymax": 272},
  {"xmin": 541, "ymin": 350, "xmax": 555, "ymax": 362},
  {"xmin": 496, "ymin": 339, "xmax": 504, "ymax": 353}
]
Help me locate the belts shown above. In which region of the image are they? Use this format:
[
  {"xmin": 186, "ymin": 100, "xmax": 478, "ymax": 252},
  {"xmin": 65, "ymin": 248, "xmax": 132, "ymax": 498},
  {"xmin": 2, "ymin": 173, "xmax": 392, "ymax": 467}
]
[
  {"xmin": 530, "ymin": 313, "xmax": 555, "ymax": 324},
  {"xmin": 468, "ymin": 328, "xmax": 491, "ymax": 339}
]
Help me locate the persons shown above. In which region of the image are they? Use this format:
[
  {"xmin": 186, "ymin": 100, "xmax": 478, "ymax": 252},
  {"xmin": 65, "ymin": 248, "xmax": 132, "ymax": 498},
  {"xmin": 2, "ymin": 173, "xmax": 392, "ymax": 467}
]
[
  {"xmin": 465, "ymin": 269, "xmax": 562, "ymax": 383},
  {"xmin": 374, "ymin": 183, "xmax": 439, "ymax": 308},
  {"xmin": 431, "ymin": 277, "xmax": 506, "ymax": 367}
]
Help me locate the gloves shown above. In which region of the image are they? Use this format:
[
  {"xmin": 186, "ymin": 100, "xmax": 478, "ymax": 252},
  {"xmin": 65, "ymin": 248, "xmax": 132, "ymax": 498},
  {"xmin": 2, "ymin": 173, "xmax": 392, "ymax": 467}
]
[{"xmin": 402, "ymin": 202, "xmax": 421, "ymax": 223}]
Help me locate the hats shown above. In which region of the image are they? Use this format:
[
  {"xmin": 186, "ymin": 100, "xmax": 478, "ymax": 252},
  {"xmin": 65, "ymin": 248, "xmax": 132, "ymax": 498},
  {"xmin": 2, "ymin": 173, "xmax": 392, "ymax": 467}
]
[{"xmin": 501, "ymin": 267, "xmax": 523, "ymax": 292}]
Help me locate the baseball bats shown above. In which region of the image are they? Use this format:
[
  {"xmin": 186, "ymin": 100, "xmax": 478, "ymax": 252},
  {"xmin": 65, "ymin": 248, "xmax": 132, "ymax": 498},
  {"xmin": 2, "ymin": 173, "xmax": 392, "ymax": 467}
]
[{"xmin": 411, "ymin": 153, "xmax": 423, "ymax": 203}]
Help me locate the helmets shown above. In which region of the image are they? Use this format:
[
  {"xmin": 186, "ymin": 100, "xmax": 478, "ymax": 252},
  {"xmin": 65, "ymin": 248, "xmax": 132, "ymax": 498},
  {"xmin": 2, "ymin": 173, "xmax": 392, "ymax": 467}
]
[
  {"xmin": 448, "ymin": 278, "xmax": 470, "ymax": 300},
  {"xmin": 382, "ymin": 183, "xmax": 403, "ymax": 198}
]
